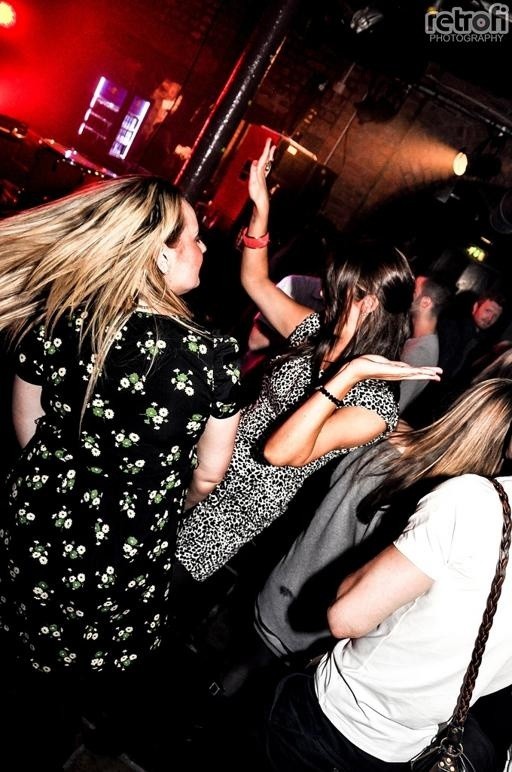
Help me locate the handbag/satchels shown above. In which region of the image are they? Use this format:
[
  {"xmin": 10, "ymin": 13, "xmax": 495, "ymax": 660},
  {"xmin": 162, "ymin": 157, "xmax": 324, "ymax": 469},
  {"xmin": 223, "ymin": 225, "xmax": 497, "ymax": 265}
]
[{"xmin": 411, "ymin": 712, "xmax": 500, "ymax": 772}]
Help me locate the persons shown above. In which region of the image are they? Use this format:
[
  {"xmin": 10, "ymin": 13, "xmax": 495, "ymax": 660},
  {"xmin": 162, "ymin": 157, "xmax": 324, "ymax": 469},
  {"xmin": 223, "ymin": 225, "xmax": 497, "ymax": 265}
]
[
  {"xmin": 1, "ymin": 170, "xmax": 244, "ymax": 770},
  {"xmin": 85, "ymin": 136, "xmax": 511, "ymax": 769}
]
